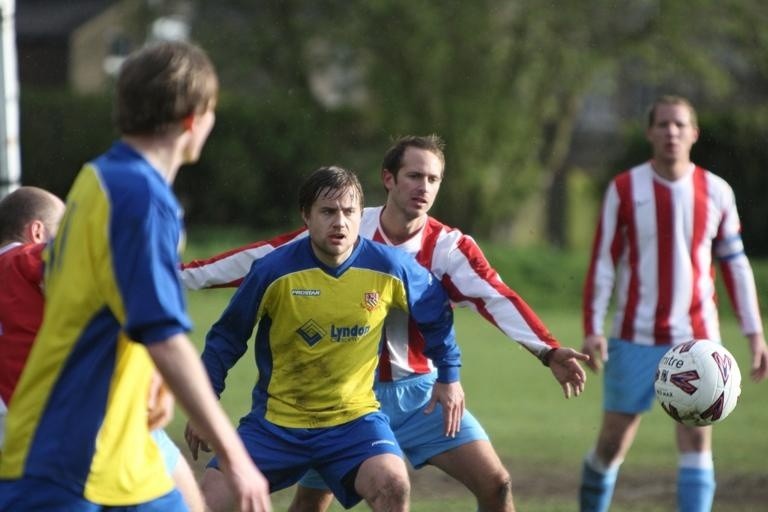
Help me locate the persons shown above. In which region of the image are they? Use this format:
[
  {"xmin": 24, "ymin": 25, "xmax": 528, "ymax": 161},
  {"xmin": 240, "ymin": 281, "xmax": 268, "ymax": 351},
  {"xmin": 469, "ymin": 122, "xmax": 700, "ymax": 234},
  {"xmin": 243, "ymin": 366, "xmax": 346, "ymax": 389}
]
[
  {"xmin": 182, "ymin": 162, "xmax": 464, "ymax": 512},
  {"xmin": 0, "ymin": 38, "xmax": 272, "ymax": 511},
  {"xmin": 575, "ymin": 92, "xmax": 767, "ymax": 512},
  {"xmin": 179, "ymin": 129, "xmax": 591, "ymax": 512},
  {"xmin": 0, "ymin": 186, "xmax": 207, "ymax": 511}
]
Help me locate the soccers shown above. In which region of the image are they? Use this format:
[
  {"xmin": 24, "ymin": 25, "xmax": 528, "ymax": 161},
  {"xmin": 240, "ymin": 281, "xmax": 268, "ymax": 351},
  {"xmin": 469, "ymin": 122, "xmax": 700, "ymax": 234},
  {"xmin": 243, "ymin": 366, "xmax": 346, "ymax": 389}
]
[{"xmin": 655, "ymin": 340, "xmax": 741, "ymax": 427}]
